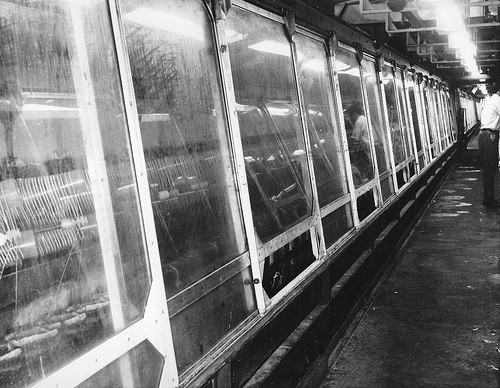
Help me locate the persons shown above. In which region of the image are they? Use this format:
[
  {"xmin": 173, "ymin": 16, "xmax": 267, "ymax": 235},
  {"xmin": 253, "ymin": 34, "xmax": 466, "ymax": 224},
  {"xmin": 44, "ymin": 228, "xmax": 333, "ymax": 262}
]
[
  {"xmin": 477, "ymin": 82, "xmax": 500, "ymax": 208},
  {"xmin": 347, "ymin": 105, "xmax": 378, "ymax": 151}
]
